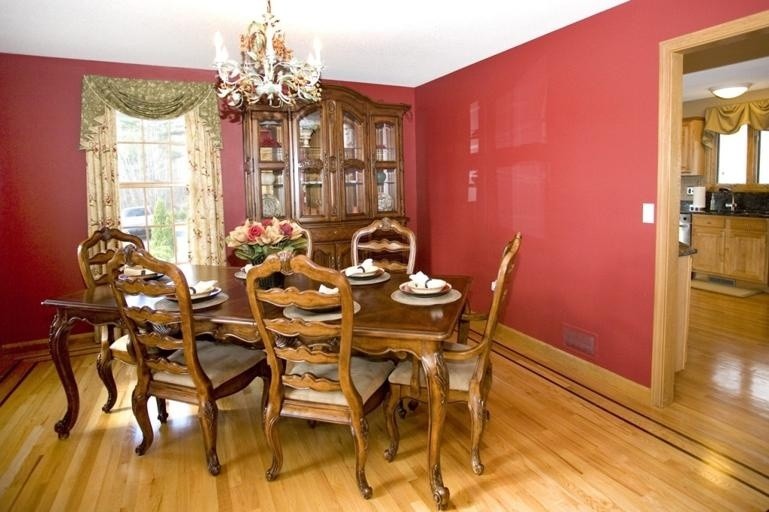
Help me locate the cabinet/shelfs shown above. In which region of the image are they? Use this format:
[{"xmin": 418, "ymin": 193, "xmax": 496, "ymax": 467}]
[
  {"xmin": 688, "ymin": 212, "xmax": 769, "ymax": 292},
  {"xmin": 670, "ymin": 241, "xmax": 697, "ymax": 372},
  {"xmin": 680, "ymin": 116, "xmax": 707, "ymax": 176},
  {"xmin": 240, "ymin": 84, "xmax": 413, "ymax": 271}
]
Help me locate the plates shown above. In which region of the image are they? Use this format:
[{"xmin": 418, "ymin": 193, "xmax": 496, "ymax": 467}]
[
  {"xmin": 340, "ymin": 268, "xmax": 385, "ymax": 279},
  {"xmin": 407, "ymin": 279, "xmax": 446, "ymax": 290},
  {"xmin": 399, "ymin": 281, "xmax": 452, "ymax": 297},
  {"xmin": 165, "ymin": 287, "xmax": 222, "ymax": 304},
  {"xmin": 117, "ymin": 272, "xmax": 164, "ymax": 281},
  {"xmin": 297, "ymin": 306, "xmax": 342, "ymax": 314}
]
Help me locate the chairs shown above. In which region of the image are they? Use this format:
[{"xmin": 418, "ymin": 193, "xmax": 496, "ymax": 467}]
[
  {"xmin": 244, "ymin": 249, "xmax": 398, "ymax": 501},
  {"xmin": 349, "ymin": 216, "xmax": 417, "ymax": 420},
  {"xmin": 76, "ymin": 226, "xmax": 172, "ymax": 426},
  {"xmin": 382, "ymin": 231, "xmax": 521, "ymax": 476},
  {"xmin": 105, "ymin": 243, "xmax": 270, "ymax": 476},
  {"xmin": 276, "ymin": 221, "xmax": 313, "ymax": 262}
]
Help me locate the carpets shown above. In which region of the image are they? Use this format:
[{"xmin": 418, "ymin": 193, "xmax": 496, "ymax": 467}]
[{"xmin": 691, "ymin": 273, "xmax": 762, "ymax": 298}]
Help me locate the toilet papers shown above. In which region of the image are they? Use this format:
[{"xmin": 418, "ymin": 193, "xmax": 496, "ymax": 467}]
[{"xmin": 693, "ymin": 187, "xmax": 706, "ymax": 207}]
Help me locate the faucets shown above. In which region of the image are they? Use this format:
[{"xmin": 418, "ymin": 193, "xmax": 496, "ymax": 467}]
[{"xmin": 720, "ymin": 188, "xmax": 737, "ymax": 211}]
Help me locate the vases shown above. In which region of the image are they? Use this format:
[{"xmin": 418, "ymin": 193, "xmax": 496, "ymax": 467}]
[{"xmin": 250, "ymin": 255, "xmax": 285, "ymax": 288}]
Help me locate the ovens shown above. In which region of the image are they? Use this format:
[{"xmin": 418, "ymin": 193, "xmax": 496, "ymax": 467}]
[{"xmin": 679, "ymin": 214, "xmax": 691, "ymax": 246}]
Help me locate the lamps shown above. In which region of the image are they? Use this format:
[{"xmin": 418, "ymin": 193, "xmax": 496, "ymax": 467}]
[
  {"xmin": 211, "ymin": 1, "xmax": 324, "ymax": 111},
  {"xmin": 709, "ymin": 85, "xmax": 750, "ymax": 100}
]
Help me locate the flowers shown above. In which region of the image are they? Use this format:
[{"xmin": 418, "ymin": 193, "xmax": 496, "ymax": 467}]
[{"xmin": 223, "ymin": 215, "xmax": 309, "ymax": 259}]
